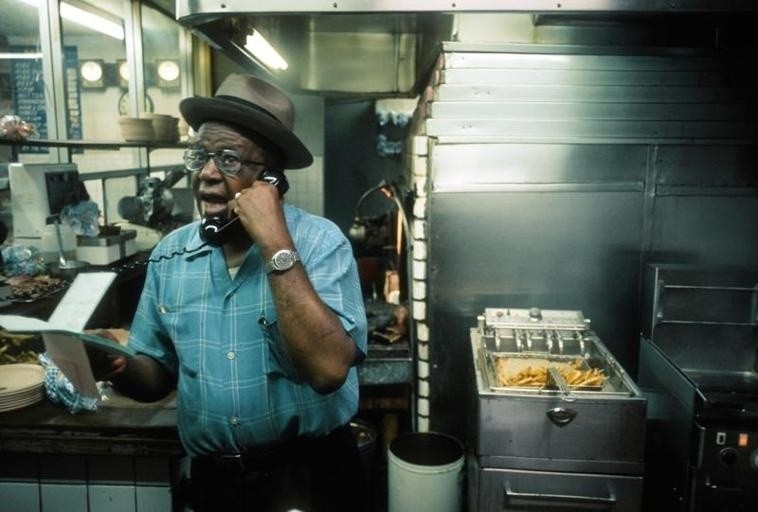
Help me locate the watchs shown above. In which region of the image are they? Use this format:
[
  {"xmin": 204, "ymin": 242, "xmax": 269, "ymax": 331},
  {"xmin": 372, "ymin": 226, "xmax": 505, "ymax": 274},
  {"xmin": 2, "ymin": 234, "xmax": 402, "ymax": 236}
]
[{"xmin": 265, "ymin": 249, "xmax": 300, "ymax": 276}]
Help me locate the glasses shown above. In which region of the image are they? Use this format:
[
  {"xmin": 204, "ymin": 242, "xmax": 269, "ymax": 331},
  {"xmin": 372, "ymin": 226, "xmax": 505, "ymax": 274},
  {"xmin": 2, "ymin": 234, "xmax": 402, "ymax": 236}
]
[{"xmin": 181, "ymin": 144, "xmax": 270, "ymax": 173}]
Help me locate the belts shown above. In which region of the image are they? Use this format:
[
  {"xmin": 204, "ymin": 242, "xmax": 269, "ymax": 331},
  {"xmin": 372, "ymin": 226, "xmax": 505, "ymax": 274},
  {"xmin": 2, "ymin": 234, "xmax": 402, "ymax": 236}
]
[{"xmin": 192, "ymin": 423, "xmax": 352, "ymax": 474}]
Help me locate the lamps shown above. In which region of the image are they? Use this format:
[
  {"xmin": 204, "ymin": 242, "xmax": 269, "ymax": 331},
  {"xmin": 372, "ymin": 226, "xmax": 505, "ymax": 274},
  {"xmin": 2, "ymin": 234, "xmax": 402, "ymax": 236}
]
[{"xmin": 231, "ymin": 24, "xmax": 288, "ymax": 74}]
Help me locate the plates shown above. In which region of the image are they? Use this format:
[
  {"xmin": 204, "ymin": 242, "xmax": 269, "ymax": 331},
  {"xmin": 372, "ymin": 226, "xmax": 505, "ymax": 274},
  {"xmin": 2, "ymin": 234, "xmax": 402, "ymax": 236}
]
[
  {"xmin": 118, "ymin": 116, "xmax": 156, "ymax": 145},
  {"xmin": 138, "ymin": 112, "xmax": 176, "ymax": 142},
  {"xmin": 1, "ymin": 365, "xmax": 47, "ymax": 413}
]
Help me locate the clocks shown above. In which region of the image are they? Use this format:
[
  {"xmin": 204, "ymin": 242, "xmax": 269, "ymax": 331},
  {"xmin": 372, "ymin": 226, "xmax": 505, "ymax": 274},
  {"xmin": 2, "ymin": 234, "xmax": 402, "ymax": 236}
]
[{"xmin": 118, "ymin": 89, "xmax": 153, "ymax": 116}]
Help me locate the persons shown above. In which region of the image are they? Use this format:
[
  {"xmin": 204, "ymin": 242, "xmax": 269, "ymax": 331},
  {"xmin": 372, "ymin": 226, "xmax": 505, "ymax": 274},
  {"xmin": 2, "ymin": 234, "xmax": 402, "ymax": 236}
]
[{"xmin": 86, "ymin": 71, "xmax": 369, "ymax": 512}]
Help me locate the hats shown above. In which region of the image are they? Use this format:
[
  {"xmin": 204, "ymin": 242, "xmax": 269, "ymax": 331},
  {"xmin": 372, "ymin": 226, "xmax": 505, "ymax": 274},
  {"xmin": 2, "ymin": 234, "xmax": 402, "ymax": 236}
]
[{"xmin": 179, "ymin": 73, "xmax": 314, "ymax": 171}]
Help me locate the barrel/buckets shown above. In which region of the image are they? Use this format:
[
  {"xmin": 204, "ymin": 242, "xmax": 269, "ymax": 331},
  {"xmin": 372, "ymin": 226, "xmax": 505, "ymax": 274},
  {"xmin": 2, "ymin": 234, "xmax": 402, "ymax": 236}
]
[{"xmin": 389, "ymin": 431, "xmax": 466, "ymax": 512}]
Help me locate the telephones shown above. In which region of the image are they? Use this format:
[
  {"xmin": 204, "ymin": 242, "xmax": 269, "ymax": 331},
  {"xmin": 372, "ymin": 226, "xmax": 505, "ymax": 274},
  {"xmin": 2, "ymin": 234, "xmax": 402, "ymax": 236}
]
[{"xmin": 199, "ymin": 167, "xmax": 291, "ymax": 248}]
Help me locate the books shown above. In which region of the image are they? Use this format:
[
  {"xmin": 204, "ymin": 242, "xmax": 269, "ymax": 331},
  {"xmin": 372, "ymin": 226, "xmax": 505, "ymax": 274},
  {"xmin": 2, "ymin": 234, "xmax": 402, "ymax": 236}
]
[{"xmin": 0, "ymin": 271, "xmax": 135, "ymax": 400}]
